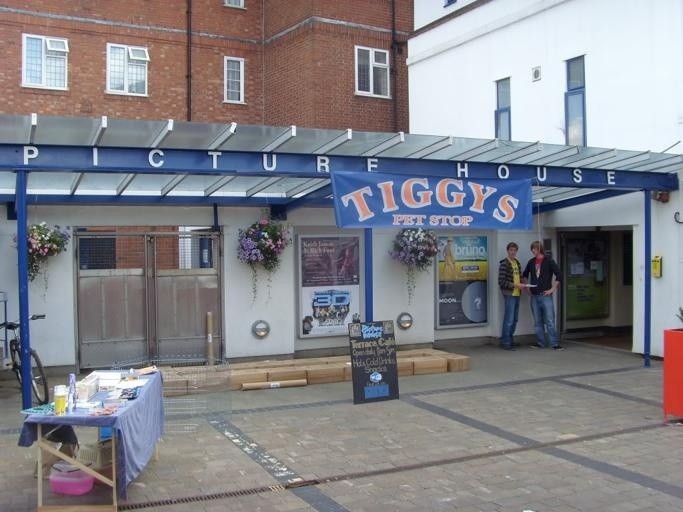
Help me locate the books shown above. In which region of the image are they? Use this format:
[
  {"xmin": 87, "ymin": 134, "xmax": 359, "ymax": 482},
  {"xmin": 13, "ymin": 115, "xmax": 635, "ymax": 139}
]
[{"xmin": 102, "ymin": 399, "xmax": 128, "ymax": 407}]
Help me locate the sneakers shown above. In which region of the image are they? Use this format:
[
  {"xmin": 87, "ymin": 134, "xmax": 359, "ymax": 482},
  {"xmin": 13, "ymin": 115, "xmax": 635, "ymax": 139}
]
[
  {"xmin": 511, "ymin": 343, "xmax": 520, "ymax": 346},
  {"xmin": 500, "ymin": 343, "xmax": 517, "ymax": 351},
  {"xmin": 552, "ymin": 345, "xmax": 565, "ymax": 351},
  {"xmin": 528, "ymin": 343, "xmax": 544, "ymax": 349}
]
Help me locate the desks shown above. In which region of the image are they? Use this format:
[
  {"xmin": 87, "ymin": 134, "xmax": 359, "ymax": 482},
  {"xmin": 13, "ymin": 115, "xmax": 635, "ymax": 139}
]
[{"xmin": 17, "ymin": 369, "xmax": 165, "ymax": 512}]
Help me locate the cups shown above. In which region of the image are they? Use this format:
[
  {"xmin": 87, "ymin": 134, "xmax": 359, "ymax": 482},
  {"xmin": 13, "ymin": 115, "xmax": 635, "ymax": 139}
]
[{"xmin": 53, "ymin": 385, "xmax": 67, "ymax": 416}]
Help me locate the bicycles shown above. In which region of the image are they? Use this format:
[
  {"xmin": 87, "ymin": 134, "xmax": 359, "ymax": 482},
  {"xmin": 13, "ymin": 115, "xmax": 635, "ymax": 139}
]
[{"xmin": 0, "ymin": 312, "xmax": 50, "ymax": 404}]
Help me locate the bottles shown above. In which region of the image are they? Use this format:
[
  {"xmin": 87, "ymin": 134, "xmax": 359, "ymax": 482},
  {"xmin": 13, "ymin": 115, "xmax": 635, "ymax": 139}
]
[{"xmin": 67, "ymin": 374, "xmax": 76, "ymax": 414}]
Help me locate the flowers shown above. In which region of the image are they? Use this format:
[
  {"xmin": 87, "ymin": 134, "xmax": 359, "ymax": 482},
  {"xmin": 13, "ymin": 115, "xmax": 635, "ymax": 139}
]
[
  {"xmin": 388, "ymin": 229, "xmax": 441, "ymax": 304},
  {"xmin": 12, "ymin": 221, "xmax": 70, "ymax": 302},
  {"xmin": 237, "ymin": 211, "xmax": 293, "ymax": 303}
]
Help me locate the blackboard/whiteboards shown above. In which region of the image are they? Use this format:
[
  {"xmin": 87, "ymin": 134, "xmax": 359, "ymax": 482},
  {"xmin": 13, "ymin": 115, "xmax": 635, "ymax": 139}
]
[{"xmin": 348, "ymin": 321, "xmax": 399, "ymax": 405}]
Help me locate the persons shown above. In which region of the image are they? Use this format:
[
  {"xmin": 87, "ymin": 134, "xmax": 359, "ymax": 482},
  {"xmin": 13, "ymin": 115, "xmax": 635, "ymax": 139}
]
[
  {"xmin": 522, "ymin": 240, "xmax": 566, "ymax": 352},
  {"xmin": 442, "ymin": 238, "xmax": 457, "ymax": 277},
  {"xmin": 498, "ymin": 241, "xmax": 523, "ymax": 350}
]
